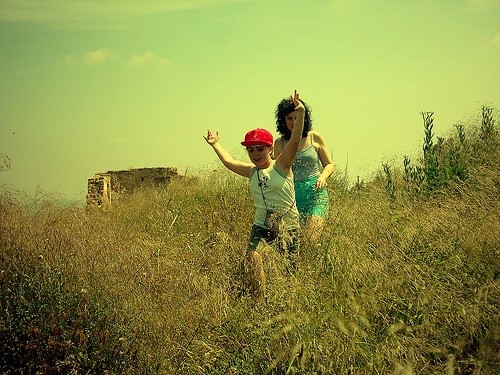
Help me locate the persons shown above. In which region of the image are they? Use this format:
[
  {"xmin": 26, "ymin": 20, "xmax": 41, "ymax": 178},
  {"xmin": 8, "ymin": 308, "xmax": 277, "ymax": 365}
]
[
  {"xmin": 203, "ymin": 90, "xmax": 312, "ymax": 297},
  {"xmin": 273, "ymin": 96, "xmax": 336, "ymax": 247}
]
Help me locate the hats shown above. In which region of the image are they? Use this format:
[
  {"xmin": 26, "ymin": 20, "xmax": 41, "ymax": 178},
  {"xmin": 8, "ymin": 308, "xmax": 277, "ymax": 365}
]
[{"xmin": 240, "ymin": 129, "xmax": 272, "ymax": 146}]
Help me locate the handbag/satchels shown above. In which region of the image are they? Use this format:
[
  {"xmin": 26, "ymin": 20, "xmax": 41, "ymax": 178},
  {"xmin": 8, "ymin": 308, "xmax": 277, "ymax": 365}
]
[{"xmin": 264, "ymin": 210, "xmax": 280, "ymax": 228}]
